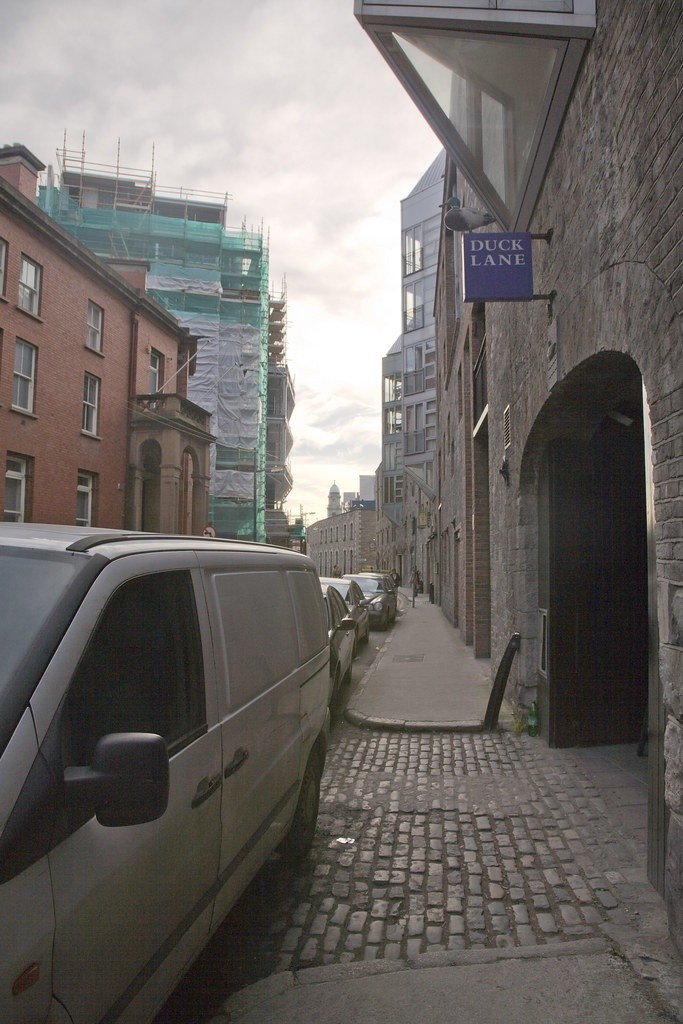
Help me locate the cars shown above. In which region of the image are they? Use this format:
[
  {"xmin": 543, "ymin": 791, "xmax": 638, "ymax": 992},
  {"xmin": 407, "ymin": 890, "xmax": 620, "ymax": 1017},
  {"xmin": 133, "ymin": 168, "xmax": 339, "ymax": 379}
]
[
  {"xmin": 343, "ymin": 572, "xmax": 395, "ymax": 631},
  {"xmin": 318, "ymin": 585, "xmax": 358, "ymax": 688},
  {"xmin": 318, "ymin": 577, "xmax": 375, "ymax": 645}
]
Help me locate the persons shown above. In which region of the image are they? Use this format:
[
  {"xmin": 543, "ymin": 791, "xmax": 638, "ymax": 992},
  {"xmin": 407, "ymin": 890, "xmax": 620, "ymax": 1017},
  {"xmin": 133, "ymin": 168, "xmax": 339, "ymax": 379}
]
[
  {"xmin": 390, "ymin": 569, "xmax": 400, "ymax": 588},
  {"xmin": 332, "ymin": 564, "xmax": 342, "ymax": 578}
]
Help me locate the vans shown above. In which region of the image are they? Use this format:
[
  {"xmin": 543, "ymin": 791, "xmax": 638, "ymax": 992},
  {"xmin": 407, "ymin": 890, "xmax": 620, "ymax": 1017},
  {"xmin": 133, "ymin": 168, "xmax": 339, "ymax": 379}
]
[{"xmin": 1, "ymin": 523, "xmax": 338, "ymax": 1024}]
